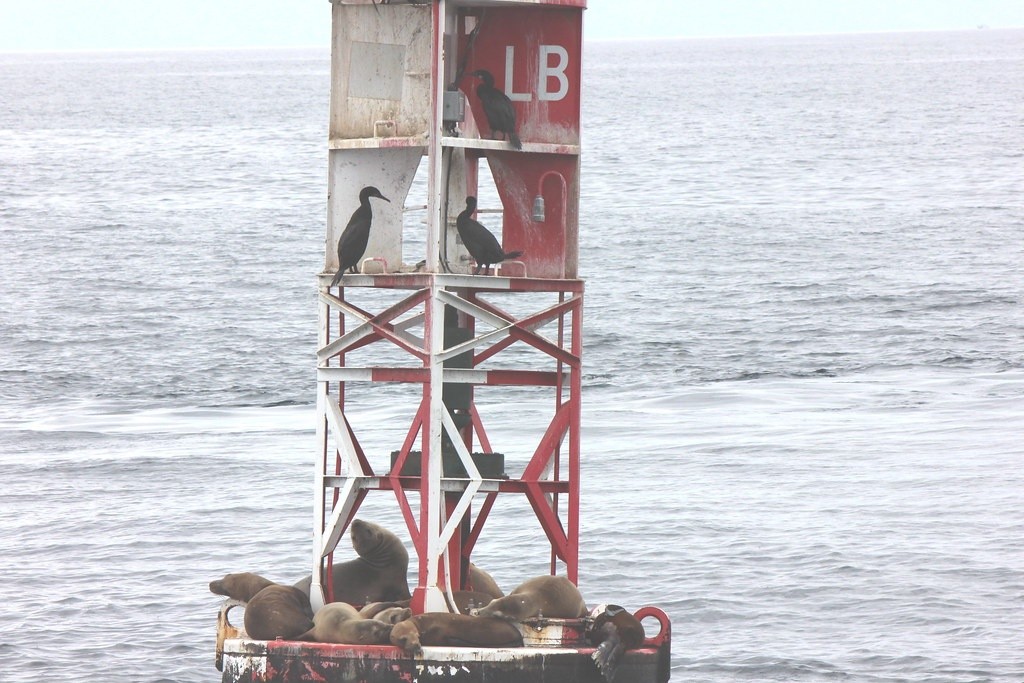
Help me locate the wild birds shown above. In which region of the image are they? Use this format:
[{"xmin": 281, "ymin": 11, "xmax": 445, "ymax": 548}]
[
  {"xmin": 457, "ymin": 196, "xmax": 521, "ymax": 275},
  {"xmin": 466, "ymin": 69, "xmax": 522, "ymax": 152},
  {"xmin": 331, "ymin": 186, "xmax": 390, "ymax": 287}
]
